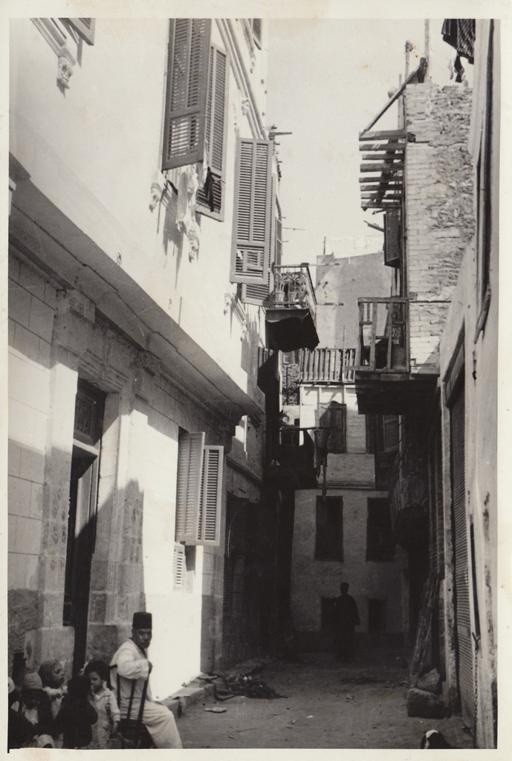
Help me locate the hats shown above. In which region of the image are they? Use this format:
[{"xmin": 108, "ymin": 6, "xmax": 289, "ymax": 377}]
[{"xmin": 132, "ymin": 611, "xmax": 152, "ymax": 630}]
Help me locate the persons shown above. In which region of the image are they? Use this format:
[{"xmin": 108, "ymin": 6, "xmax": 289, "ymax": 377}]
[
  {"xmin": 333, "ymin": 583, "xmax": 360, "ymax": 662},
  {"xmin": 8, "ymin": 612, "xmax": 183, "ymax": 748}
]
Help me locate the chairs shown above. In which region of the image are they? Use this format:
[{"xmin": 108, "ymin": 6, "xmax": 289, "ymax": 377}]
[{"xmin": 106, "ymin": 664, "xmax": 149, "ymax": 749}]
